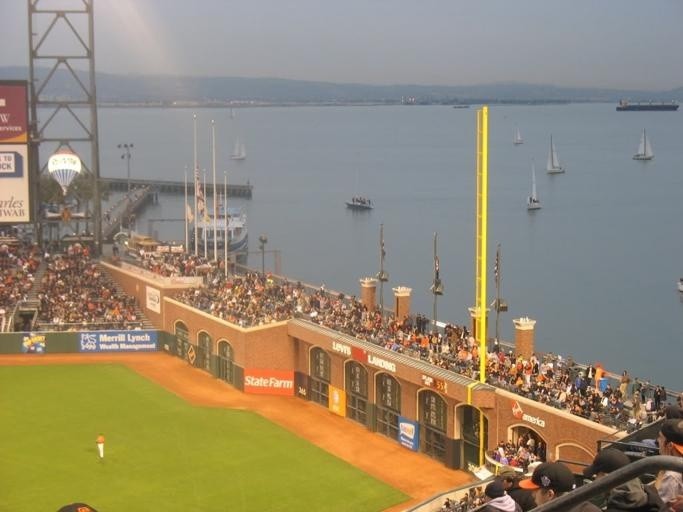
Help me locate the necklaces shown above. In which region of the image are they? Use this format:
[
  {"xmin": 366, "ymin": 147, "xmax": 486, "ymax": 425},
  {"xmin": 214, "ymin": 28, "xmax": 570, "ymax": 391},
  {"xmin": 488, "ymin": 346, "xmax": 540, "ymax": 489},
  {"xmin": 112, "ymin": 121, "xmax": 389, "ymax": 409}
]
[
  {"xmin": 526, "ymin": 159, "xmax": 542, "ymax": 210},
  {"xmin": 513, "ymin": 127, "xmax": 524, "ymax": 143},
  {"xmin": 633, "ymin": 128, "xmax": 653, "ymax": 161},
  {"xmin": 232, "ymin": 136, "xmax": 246, "ymax": 161},
  {"xmin": 548, "ymin": 134, "xmax": 565, "ymax": 174}
]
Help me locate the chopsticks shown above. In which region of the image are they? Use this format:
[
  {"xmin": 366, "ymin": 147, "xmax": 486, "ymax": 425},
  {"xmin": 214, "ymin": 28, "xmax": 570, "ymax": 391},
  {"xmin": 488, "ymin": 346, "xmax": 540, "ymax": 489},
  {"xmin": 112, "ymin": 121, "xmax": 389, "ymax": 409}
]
[{"xmin": 47, "ymin": 149, "xmax": 81, "ymax": 196}]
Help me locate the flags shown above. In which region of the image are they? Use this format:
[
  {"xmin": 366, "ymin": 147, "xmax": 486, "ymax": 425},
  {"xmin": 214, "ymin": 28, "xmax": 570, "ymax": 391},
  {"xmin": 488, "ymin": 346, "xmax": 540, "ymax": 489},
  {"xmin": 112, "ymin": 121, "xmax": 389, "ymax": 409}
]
[
  {"xmin": 196, "ymin": 166, "xmax": 210, "ymax": 223},
  {"xmin": 186, "ymin": 205, "xmax": 194, "ymax": 223}
]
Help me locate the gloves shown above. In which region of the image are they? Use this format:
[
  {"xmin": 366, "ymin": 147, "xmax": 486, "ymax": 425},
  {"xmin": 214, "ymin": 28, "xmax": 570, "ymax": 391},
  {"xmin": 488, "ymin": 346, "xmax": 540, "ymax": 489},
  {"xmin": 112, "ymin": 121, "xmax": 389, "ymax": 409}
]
[
  {"xmin": 124, "ymin": 231, "xmax": 164, "ymax": 261},
  {"xmin": 346, "ymin": 200, "xmax": 375, "ymax": 208},
  {"xmin": 615, "ymin": 99, "xmax": 680, "ymax": 111},
  {"xmin": 190, "ymin": 203, "xmax": 248, "ymax": 259}
]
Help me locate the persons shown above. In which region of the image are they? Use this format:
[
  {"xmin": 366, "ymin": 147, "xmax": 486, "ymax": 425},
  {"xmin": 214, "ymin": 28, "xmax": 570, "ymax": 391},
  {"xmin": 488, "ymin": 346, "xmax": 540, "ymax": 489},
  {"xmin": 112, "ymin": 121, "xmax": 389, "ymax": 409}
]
[
  {"xmin": 584, "ymin": 448, "xmax": 649, "ymax": 512},
  {"xmin": 444, "ymin": 486, "xmax": 485, "ymax": 512},
  {"xmin": 485, "ymin": 483, "xmax": 522, "ymax": 512},
  {"xmin": 504, "ymin": 478, "xmax": 537, "ymax": 512},
  {"xmin": 648, "ymin": 419, "xmax": 683, "ymax": 512},
  {"xmin": 494, "ymin": 432, "xmax": 545, "ymax": 469},
  {"xmin": 95, "ymin": 433, "xmax": 105, "ymax": 458},
  {"xmin": 103, "ymin": 192, "xmax": 138, "ymax": 222},
  {"xmin": 519, "ymin": 462, "xmax": 601, "ymax": 512},
  {"xmin": 0, "ymin": 197, "xmax": 683, "ymax": 432}
]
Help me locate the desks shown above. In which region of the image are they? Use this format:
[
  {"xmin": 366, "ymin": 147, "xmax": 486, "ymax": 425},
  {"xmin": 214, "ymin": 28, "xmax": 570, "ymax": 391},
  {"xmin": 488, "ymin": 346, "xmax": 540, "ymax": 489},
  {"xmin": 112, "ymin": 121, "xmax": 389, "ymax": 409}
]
[
  {"xmin": 430, "ymin": 232, "xmax": 444, "ymax": 336},
  {"xmin": 377, "ymin": 224, "xmax": 388, "ymax": 314},
  {"xmin": 491, "ymin": 243, "xmax": 507, "ymax": 353},
  {"xmin": 117, "ymin": 142, "xmax": 133, "ymax": 190}
]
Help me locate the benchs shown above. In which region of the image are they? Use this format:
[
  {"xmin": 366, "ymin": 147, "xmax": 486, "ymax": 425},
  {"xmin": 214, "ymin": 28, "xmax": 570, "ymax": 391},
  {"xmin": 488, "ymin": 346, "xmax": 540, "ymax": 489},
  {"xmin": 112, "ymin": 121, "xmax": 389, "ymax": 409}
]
[
  {"xmin": 186, "ymin": 205, "xmax": 194, "ymax": 223},
  {"xmin": 196, "ymin": 166, "xmax": 210, "ymax": 223}
]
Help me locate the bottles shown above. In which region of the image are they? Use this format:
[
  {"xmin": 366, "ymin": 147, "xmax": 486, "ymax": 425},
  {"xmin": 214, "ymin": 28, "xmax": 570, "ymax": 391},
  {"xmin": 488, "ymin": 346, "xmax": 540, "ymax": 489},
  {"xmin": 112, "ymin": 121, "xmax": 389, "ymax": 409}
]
[
  {"xmin": 660, "ymin": 418, "xmax": 683, "ymax": 455},
  {"xmin": 583, "ymin": 448, "xmax": 631, "ymax": 478},
  {"xmin": 484, "ymin": 461, "xmax": 576, "ymax": 498}
]
